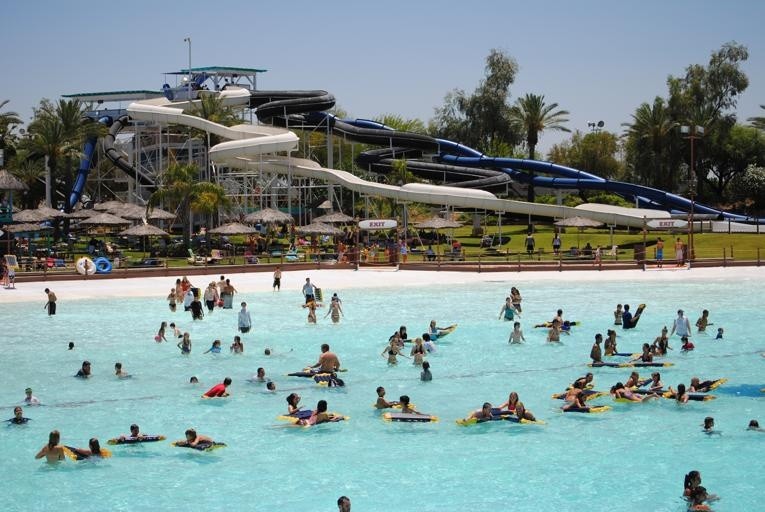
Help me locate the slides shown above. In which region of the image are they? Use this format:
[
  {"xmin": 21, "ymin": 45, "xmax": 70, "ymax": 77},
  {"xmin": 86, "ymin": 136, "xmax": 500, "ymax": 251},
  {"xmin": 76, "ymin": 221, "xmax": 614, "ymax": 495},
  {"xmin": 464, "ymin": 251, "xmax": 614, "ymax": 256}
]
[
  {"xmin": 11, "ymin": 115, "xmax": 114, "ymax": 241},
  {"xmin": 126, "ymin": 80, "xmax": 673, "ymax": 230},
  {"xmin": 104, "ymin": 113, "xmax": 209, "ymax": 234},
  {"xmin": 320, "ymin": 110, "xmax": 765, "ymax": 224},
  {"xmin": 221, "ymin": 82, "xmax": 643, "ymax": 231}
]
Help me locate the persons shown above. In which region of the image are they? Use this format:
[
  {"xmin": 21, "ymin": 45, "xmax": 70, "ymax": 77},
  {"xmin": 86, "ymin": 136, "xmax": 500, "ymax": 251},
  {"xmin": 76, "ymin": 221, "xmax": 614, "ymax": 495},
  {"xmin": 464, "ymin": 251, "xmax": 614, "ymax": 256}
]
[
  {"xmin": 570, "ymin": 247, "xmax": 581, "ymax": 255},
  {"xmin": 158, "ymin": 322, "xmax": 168, "ymax": 342},
  {"xmin": 550, "ymin": 232, "xmax": 561, "ymax": 255},
  {"xmin": 267, "ymin": 381, "xmax": 275, "ymax": 390},
  {"xmin": 264, "ymin": 346, "xmax": 294, "ymax": 355},
  {"xmin": 203, "ymin": 377, "xmax": 232, "ymax": 397},
  {"xmin": 524, "ymin": 233, "xmax": 536, "ymax": 258},
  {"xmin": 230, "ymin": 336, "xmax": 243, "ymax": 353},
  {"xmin": 257, "ymin": 368, "xmax": 265, "ymax": 379},
  {"xmin": 238, "ymin": 302, "xmax": 251, "ymax": 333},
  {"xmin": 0, "ymin": 258, "xmax": 18, "ymax": 290},
  {"xmin": 682, "ymin": 471, "xmax": 719, "ymax": 498},
  {"xmin": 583, "ymin": 243, "xmax": 593, "ymax": 255},
  {"xmin": 75, "ymin": 438, "xmax": 103, "ymax": 456},
  {"xmin": 3, "ymin": 407, "xmax": 32, "ymax": 425},
  {"xmin": 674, "ymin": 237, "xmax": 686, "ymax": 267},
  {"xmin": 463, "ymin": 402, "xmax": 494, "ymax": 422},
  {"xmin": 286, "ymin": 393, "xmax": 304, "ymax": 414},
  {"xmin": 702, "ymin": 416, "xmax": 715, "ymax": 432},
  {"xmin": 657, "ymin": 237, "xmax": 665, "ymax": 268},
  {"xmin": 497, "ymin": 286, "xmax": 726, "ymax": 412},
  {"xmin": 68, "ymin": 342, "xmax": 75, "ymax": 350},
  {"xmin": 176, "ymin": 428, "xmax": 212, "ymax": 448},
  {"xmin": 375, "ymin": 386, "xmax": 396, "ymax": 410},
  {"xmin": 592, "ymin": 245, "xmax": 603, "ymax": 271},
  {"xmin": 203, "ymin": 340, "xmax": 221, "ymax": 354},
  {"xmin": 516, "ymin": 401, "xmax": 536, "ymax": 422},
  {"xmin": 75, "ymin": 361, "xmax": 91, "ymax": 378},
  {"xmin": 190, "ymin": 376, "xmax": 198, "ymax": 383},
  {"xmin": 749, "ymin": 420, "xmax": 759, "ymax": 429},
  {"xmin": 166, "ymin": 274, "xmax": 238, "ymax": 323},
  {"xmin": 400, "ymin": 396, "xmax": 427, "ymax": 416},
  {"xmin": 44, "ymin": 288, "xmax": 57, "ymax": 315},
  {"xmin": 308, "ymin": 344, "xmax": 340, "ymax": 371},
  {"xmin": 272, "ymin": 265, "xmax": 282, "ymax": 291},
  {"xmin": 121, "ymin": 424, "xmax": 145, "ymax": 442},
  {"xmin": 687, "ymin": 486, "xmax": 714, "ymax": 512},
  {"xmin": 170, "ymin": 323, "xmax": 183, "ymax": 338},
  {"xmin": 380, "ymin": 318, "xmax": 459, "ymax": 382},
  {"xmin": 299, "ymin": 276, "xmax": 346, "ymax": 324},
  {"xmin": 35, "ymin": 431, "xmax": 65, "ymax": 461},
  {"xmin": 19, "ymin": 387, "xmax": 39, "ymax": 403},
  {"xmin": 177, "ymin": 332, "xmax": 192, "ymax": 354},
  {"xmin": 336, "ymin": 496, "xmax": 351, "ymax": 511},
  {"xmin": 312, "ymin": 400, "xmax": 342, "ymax": 424},
  {"xmin": 111, "ymin": 363, "xmax": 128, "ymax": 377}
]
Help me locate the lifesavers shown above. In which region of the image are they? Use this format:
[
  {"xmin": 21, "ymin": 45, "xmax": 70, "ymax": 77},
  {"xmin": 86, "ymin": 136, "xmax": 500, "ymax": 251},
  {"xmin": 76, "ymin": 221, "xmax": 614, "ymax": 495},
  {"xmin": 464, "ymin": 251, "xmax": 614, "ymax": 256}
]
[{"xmin": 76, "ymin": 255, "xmax": 112, "ymax": 275}]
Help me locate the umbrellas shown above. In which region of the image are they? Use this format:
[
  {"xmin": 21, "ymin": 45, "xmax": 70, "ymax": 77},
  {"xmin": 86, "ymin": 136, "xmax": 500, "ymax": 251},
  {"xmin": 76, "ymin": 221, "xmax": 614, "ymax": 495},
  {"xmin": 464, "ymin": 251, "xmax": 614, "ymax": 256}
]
[{"xmin": 553, "ymin": 215, "xmax": 603, "ymax": 247}]
